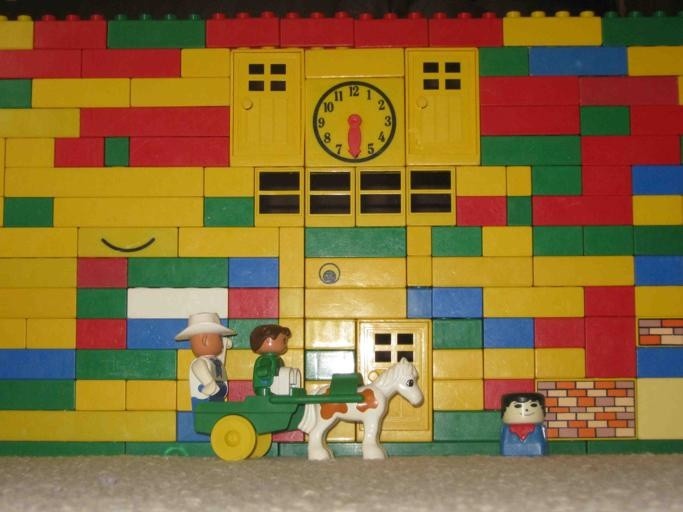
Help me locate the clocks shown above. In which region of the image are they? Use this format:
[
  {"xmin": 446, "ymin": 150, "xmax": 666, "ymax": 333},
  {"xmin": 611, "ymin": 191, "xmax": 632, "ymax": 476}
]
[{"xmin": 311, "ymin": 79, "xmax": 396, "ymax": 164}]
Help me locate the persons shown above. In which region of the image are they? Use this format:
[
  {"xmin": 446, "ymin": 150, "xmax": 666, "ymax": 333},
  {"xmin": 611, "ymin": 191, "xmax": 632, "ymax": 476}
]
[{"xmin": 494, "ymin": 390, "xmax": 550, "ymax": 458}]
